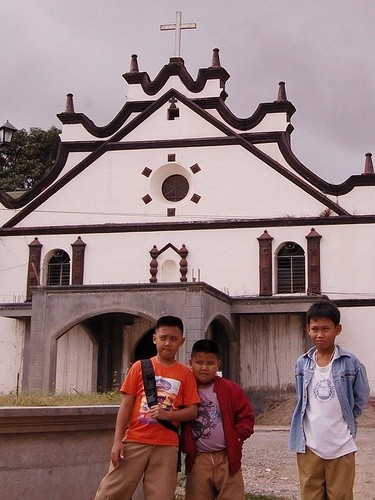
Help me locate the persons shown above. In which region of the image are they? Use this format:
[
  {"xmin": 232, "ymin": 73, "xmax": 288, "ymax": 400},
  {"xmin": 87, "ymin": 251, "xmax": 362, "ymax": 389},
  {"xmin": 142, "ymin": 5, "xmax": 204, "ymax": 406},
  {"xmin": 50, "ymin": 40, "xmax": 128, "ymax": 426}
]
[
  {"xmin": 176, "ymin": 339, "xmax": 256, "ymax": 500},
  {"xmin": 92, "ymin": 316, "xmax": 202, "ymax": 499},
  {"xmin": 287, "ymin": 299, "xmax": 371, "ymax": 500}
]
